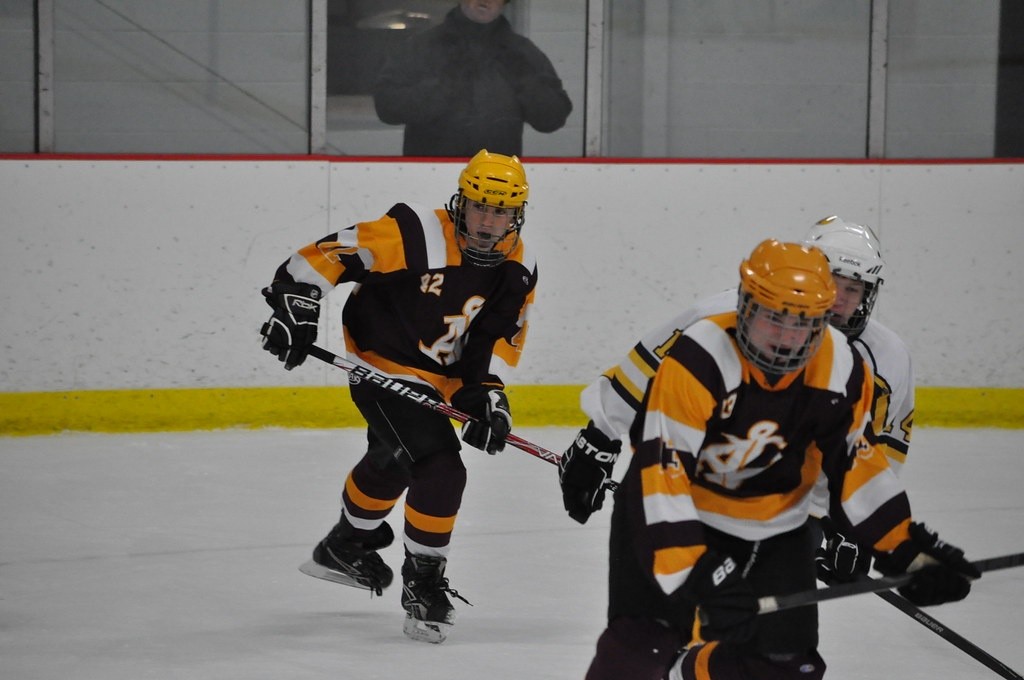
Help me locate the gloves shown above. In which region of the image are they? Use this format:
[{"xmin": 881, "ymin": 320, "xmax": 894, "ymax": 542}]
[
  {"xmin": 261, "ymin": 281, "xmax": 321, "ymax": 370},
  {"xmin": 815, "ymin": 518, "xmax": 872, "ymax": 587},
  {"xmin": 875, "ymin": 522, "xmax": 981, "ymax": 607},
  {"xmin": 557, "ymin": 420, "xmax": 621, "ymax": 524},
  {"xmin": 683, "ymin": 549, "xmax": 758, "ymax": 646},
  {"xmin": 462, "ymin": 389, "xmax": 512, "ymax": 455}
]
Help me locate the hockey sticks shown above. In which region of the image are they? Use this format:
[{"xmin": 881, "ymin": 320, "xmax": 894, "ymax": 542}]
[
  {"xmin": 259, "ymin": 322, "xmax": 621, "ymax": 493},
  {"xmin": 861, "ymin": 575, "xmax": 1024, "ymax": 680},
  {"xmin": 697, "ymin": 552, "xmax": 1024, "ymax": 626}
]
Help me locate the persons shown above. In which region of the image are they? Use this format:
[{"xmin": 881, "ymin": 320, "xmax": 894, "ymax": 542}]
[
  {"xmin": 559, "ymin": 214, "xmax": 915, "ymax": 654},
  {"xmin": 370, "ymin": 0, "xmax": 573, "ymax": 157},
  {"xmin": 586, "ymin": 239, "xmax": 981, "ymax": 680},
  {"xmin": 260, "ymin": 150, "xmax": 537, "ymax": 646}
]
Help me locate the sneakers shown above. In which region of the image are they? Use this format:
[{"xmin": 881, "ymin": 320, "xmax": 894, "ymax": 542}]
[
  {"xmin": 401, "ymin": 544, "xmax": 473, "ymax": 643},
  {"xmin": 299, "ymin": 508, "xmax": 394, "ymax": 597}
]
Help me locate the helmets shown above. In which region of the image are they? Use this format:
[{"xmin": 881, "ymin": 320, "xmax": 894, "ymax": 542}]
[
  {"xmin": 455, "ymin": 149, "xmax": 530, "ymax": 267},
  {"xmin": 807, "ymin": 215, "xmax": 885, "ymax": 342},
  {"xmin": 736, "ymin": 238, "xmax": 839, "ymax": 376}
]
